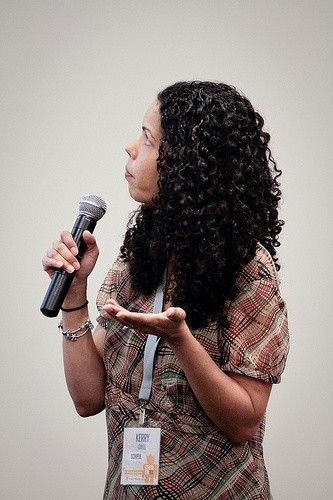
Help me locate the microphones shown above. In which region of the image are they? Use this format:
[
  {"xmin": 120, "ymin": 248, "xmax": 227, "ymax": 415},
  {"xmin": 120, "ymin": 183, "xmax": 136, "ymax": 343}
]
[{"xmin": 39, "ymin": 195, "xmax": 106, "ymax": 318}]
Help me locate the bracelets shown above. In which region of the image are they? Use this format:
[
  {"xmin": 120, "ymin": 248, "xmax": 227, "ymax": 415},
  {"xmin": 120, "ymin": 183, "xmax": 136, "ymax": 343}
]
[
  {"xmin": 58, "ymin": 319, "xmax": 94, "ymax": 342},
  {"xmin": 59, "ymin": 300, "xmax": 89, "ymax": 313}
]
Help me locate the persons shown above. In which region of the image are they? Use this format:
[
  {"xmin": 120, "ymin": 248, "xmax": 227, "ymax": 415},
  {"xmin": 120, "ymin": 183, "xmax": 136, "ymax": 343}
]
[{"xmin": 39, "ymin": 78, "xmax": 291, "ymax": 500}]
